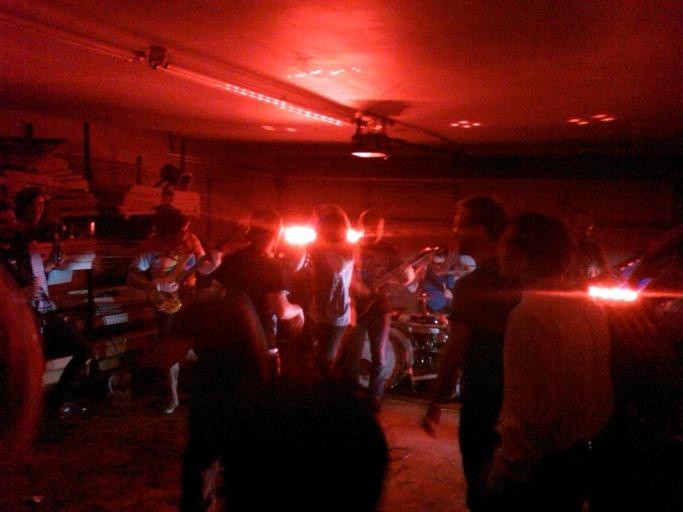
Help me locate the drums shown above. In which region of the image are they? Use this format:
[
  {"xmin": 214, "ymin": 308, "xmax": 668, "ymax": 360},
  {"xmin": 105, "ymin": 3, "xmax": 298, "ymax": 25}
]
[
  {"xmin": 406, "ymin": 325, "xmax": 441, "ymax": 368},
  {"xmin": 359, "ymin": 318, "xmax": 414, "ymax": 391}
]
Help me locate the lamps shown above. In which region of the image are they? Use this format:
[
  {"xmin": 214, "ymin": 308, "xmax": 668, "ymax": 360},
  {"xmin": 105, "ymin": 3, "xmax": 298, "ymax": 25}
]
[{"xmin": 351, "ymin": 109, "xmax": 393, "ymax": 159}]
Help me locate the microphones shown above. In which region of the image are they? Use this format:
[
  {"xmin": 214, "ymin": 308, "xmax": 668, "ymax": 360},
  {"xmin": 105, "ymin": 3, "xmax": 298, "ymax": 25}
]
[{"xmin": 407, "ymin": 367, "xmax": 414, "ymax": 386}]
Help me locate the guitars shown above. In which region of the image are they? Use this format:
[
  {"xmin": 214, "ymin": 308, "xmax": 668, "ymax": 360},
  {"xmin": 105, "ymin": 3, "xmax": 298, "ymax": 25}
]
[
  {"xmin": 150, "ymin": 217, "xmax": 251, "ymax": 314},
  {"xmin": 565, "ymin": 253, "xmax": 646, "ymax": 286},
  {"xmin": 355, "ymin": 246, "xmax": 444, "ymax": 318}
]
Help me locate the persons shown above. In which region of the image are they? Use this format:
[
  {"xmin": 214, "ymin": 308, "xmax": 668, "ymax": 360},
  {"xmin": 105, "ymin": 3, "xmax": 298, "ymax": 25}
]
[
  {"xmin": 126, "ymin": 203, "xmax": 419, "ymax": 510},
  {"xmin": 483, "ymin": 212, "xmax": 632, "ymax": 511},
  {"xmin": 420, "ymin": 197, "xmax": 523, "ymax": 510},
  {"xmin": 12, "ymin": 187, "xmax": 64, "ymax": 296}
]
